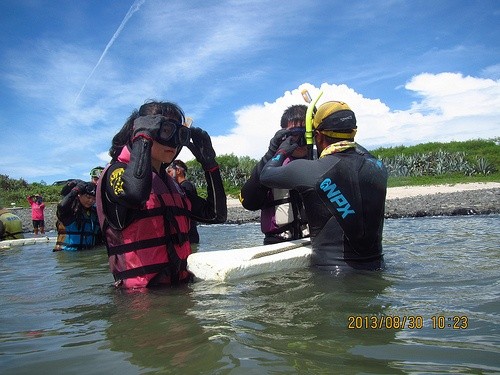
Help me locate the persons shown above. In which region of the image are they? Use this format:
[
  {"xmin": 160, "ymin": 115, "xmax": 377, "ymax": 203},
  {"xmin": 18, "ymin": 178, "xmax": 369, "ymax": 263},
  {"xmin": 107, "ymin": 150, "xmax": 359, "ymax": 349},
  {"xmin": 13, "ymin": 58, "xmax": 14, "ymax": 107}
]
[
  {"xmin": 95, "ymin": 101, "xmax": 227, "ymax": 293},
  {"xmin": 0, "ymin": 159, "xmax": 199, "ymax": 246},
  {"xmin": 238, "ymin": 100, "xmax": 389, "ymax": 272}
]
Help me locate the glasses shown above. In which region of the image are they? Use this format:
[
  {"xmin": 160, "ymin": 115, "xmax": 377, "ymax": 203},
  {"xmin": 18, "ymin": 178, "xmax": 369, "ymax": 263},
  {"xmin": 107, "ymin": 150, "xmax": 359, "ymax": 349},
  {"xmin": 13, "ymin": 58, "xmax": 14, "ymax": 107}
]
[
  {"xmin": 286, "ymin": 126, "xmax": 306, "ymax": 146},
  {"xmin": 152, "ymin": 119, "xmax": 184, "ymax": 153},
  {"xmin": 90, "ymin": 169, "xmax": 104, "ymax": 177}
]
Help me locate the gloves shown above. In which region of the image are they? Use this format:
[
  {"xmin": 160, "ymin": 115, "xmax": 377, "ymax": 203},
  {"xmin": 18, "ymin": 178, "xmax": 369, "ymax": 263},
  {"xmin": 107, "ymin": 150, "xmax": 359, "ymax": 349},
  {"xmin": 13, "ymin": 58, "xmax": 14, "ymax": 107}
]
[
  {"xmin": 130, "ymin": 113, "xmax": 168, "ymax": 143},
  {"xmin": 177, "ymin": 126, "xmax": 218, "ymax": 171},
  {"xmin": 272, "ymin": 136, "xmax": 299, "ymax": 158},
  {"xmin": 268, "ymin": 128, "xmax": 292, "ymax": 153},
  {"xmin": 72, "ymin": 180, "xmax": 88, "ymax": 194}
]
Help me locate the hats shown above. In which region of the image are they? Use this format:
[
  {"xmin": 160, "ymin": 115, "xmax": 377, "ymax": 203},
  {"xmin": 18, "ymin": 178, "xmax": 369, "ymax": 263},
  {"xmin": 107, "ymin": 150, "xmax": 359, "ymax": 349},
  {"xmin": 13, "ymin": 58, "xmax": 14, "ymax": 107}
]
[
  {"xmin": 83, "ymin": 182, "xmax": 97, "ymax": 196},
  {"xmin": 313, "ymin": 101, "xmax": 358, "ymax": 139}
]
